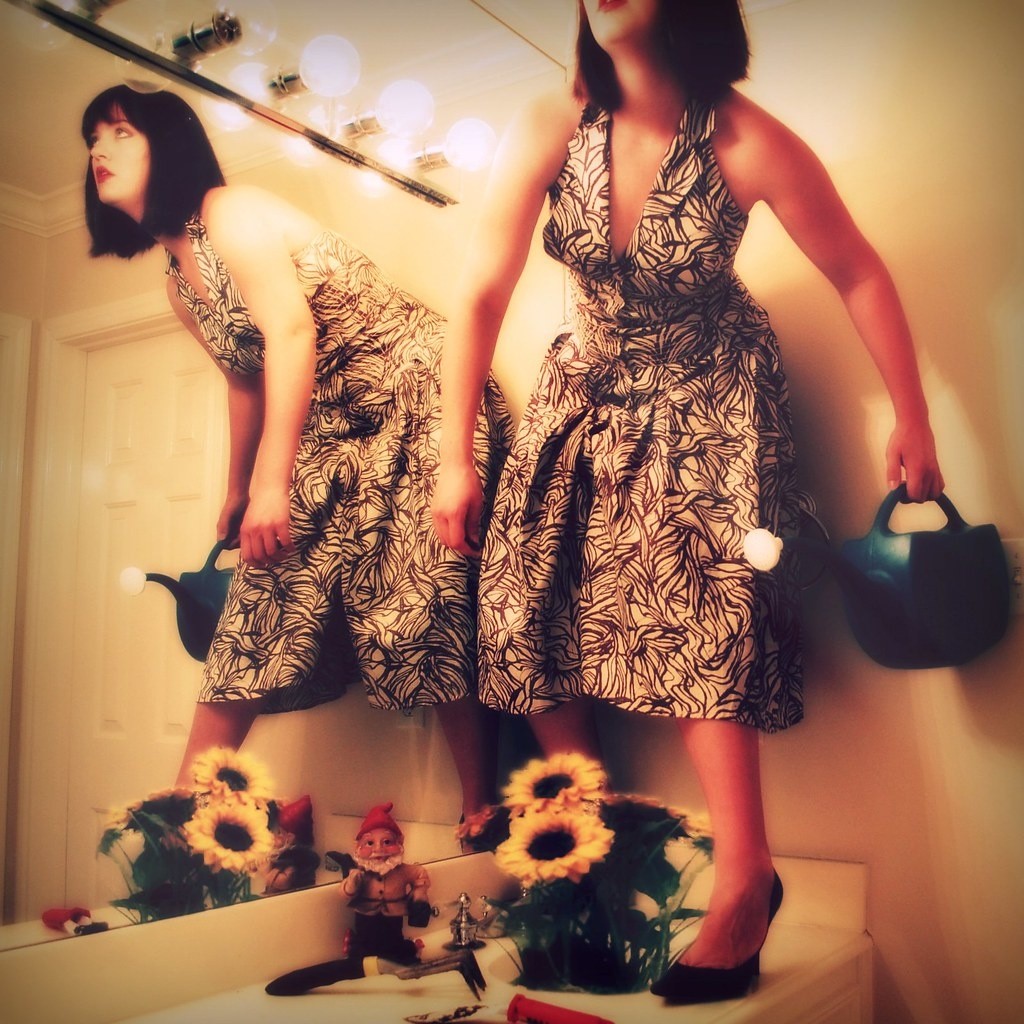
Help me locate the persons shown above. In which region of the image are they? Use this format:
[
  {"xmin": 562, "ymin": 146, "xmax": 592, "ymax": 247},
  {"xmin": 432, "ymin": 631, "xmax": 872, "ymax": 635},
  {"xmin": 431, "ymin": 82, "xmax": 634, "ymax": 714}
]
[
  {"xmin": 81, "ymin": 84, "xmax": 515, "ymax": 857},
  {"xmin": 339, "ymin": 802, "xmax": 433, "ymax": 964},
  {"xmin": 429, "ymin": 0, "xmax": 946, "ymax": 1001},
  {"xmin": 263, "ymin": 794, "xmax": 321, "ymax": 892}
]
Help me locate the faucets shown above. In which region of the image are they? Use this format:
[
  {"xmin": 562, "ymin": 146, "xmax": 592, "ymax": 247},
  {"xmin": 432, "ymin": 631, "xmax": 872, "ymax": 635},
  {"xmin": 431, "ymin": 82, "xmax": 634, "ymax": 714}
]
[{"xmin": 477, "ymin": 888, "xmax": 549, "ymax": 938}]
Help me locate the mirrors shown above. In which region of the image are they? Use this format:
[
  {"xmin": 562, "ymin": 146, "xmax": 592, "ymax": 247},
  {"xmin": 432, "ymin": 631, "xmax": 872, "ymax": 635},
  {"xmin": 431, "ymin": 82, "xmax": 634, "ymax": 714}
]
[{"xmin": 0, "ymin": 0, "xmax": 515, "ymax": 946}]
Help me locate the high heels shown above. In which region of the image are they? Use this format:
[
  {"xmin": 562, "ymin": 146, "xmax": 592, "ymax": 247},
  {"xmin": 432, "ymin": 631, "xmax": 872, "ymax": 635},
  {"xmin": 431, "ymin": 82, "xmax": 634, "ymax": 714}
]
[{"xmin": 650, "ymin": 872, "xmax": 784, "ymax": 1001}]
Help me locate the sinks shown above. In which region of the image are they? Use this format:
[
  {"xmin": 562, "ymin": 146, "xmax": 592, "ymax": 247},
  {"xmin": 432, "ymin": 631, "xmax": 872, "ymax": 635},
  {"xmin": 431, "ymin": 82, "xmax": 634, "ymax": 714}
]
[{"xmin": 486, "ymin": 919, "xmax": 679, "ymax": 999}]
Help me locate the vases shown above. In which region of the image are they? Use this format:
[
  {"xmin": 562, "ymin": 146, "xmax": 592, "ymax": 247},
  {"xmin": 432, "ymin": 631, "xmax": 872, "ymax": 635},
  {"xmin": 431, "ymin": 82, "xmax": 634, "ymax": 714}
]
[{"xmin": 500, "ymin": 875, "xmax": 699, "ymax": 997}]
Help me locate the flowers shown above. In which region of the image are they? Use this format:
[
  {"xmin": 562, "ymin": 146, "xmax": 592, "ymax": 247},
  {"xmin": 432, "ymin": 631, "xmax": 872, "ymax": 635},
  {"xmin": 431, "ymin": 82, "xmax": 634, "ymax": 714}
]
[
  {"xmin": 93, "ymin": 744, "xmax": 287, "ymax": 925},
  {"xmin": 453, "ymin": 750, "xmax": 714, "ymax": 995}
]
